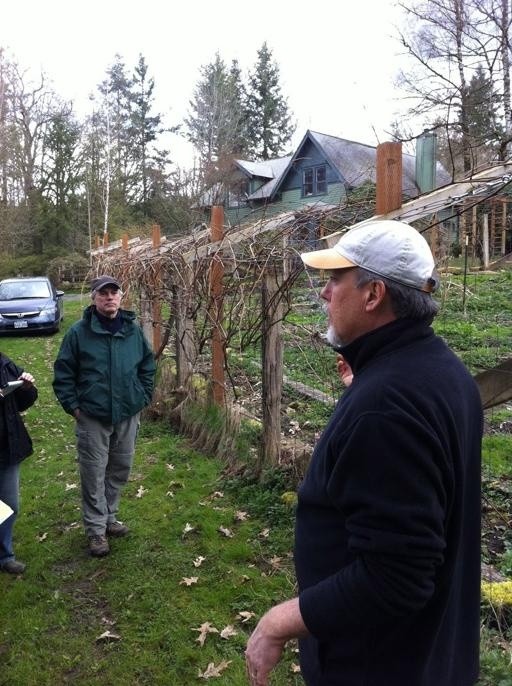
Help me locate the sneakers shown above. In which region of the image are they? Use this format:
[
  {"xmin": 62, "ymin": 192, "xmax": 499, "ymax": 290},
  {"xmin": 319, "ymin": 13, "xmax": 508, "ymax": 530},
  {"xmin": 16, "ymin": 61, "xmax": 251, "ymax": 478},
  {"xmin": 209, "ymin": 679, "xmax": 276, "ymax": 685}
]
[
  {"xmin": 88, "ymin": 535, "xmax": 110, "ymax": 558},
  {"xmin": 2, "ymin": 559, "xmax": 26, "ymax": 574},
  {"xmin": 106, "ymin": 523, "xmax": 129, "ymax": 536}
]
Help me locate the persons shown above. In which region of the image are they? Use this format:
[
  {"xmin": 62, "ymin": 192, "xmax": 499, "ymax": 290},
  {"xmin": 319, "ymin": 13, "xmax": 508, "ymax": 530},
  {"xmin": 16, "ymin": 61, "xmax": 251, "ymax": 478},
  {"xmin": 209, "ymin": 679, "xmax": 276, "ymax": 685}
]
[
  {"xmin": 241, "ymin": 216, "xmax": 485, "ymax": 686},
  {"xmin": 0, "ymin": 346, "xmax": 39, "ymax": 574},
  {"xmin": 52, "ymin": 274, "xmax": 158, "ymax": 556}
]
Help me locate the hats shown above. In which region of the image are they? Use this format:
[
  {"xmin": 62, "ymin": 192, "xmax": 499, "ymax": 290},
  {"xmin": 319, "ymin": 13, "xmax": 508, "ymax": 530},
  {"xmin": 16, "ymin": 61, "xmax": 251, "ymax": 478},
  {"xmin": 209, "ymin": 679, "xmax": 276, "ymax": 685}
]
[
  {"xmin": 90, "ymin": 275, "xmax": 121, "ymax": 293},
  {"xmin": 299, "ymin": 221, "xmax": 439, "ymax": 293}
]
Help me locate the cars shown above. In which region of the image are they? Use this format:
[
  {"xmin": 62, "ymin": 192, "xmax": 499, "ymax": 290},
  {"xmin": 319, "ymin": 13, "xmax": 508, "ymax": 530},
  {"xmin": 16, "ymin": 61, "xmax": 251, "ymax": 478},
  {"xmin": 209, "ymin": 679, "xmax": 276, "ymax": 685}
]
[{"xmin": 0, "ymin": 276, "xmax": 64, "ymax": 336}]
